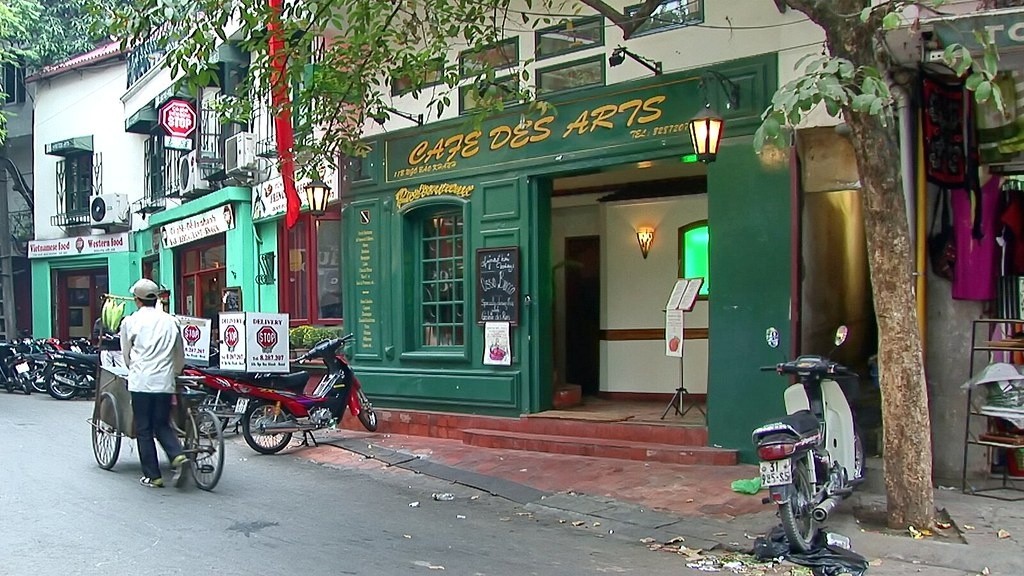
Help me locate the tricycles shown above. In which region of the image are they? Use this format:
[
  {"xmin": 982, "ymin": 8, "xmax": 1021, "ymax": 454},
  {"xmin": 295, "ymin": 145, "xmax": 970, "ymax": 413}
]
[{"xmin": 86, "ymin": 292, "xmax": 225, "ymax": 491}]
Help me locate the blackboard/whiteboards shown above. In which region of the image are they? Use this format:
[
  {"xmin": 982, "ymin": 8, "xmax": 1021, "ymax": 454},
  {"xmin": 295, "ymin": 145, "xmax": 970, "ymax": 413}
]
[
  {"xmin": 221, "ymin": 287, "xmax": 242, "ymax": 312},
  {"xmin": 476, "ymin": 246, "xmax": 520, "ymax": 327}
]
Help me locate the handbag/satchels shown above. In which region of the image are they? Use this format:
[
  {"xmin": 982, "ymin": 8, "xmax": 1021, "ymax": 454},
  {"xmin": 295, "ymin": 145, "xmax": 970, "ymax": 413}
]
[{"xmin": 931, "ymin": 227, "xmax": 958, "ymax": 280}]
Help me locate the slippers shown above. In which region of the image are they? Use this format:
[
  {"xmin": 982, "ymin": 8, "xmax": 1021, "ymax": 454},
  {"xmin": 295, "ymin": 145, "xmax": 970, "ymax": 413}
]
[
  {"xmin": 139, "ymin": 476, "xmax": 157, "ymax": 487},
  {"xmin": 172, "ymin": 458, "xmax": 190, "ymax": 486}
]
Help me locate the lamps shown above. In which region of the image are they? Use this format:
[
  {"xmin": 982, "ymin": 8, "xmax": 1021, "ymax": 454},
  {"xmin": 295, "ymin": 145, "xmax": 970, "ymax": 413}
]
[
  {"xmin": 687, "ymin": 69, "xmax": 740, "ymax": 166},
  {"xmin": 375, "ymin": 105, "xmax": 424, "ymax": 127},
  {"xmin": 478, "ymin": 79, "xmax": 518, "ymax": 101},
  {"xmin": 302, "ymin": 170, "xmax": 341, "ymax": 226},
  {"xmin": 636, "ymin": 231, "xmax": 655, "ymax": 260},
  {"xmin": 609, "ymin": 43, "xmax": 664, "ymax": 77}
]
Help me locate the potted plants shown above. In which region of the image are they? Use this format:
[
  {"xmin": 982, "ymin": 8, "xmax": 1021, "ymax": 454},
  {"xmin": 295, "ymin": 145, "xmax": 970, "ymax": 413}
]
[{"xmin": 289, "ymin": 325, "xmax": 344, "ymax": 359}]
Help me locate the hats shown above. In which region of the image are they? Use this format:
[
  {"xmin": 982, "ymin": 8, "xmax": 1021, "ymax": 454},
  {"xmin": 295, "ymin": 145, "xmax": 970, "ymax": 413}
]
[
  {"xmin": 973, "ymin": 363, "xmax": 1024, "ymax": 385},
  {"xmin": 129, "ymin": 279, "xmax": 159, "ymax": 299}
]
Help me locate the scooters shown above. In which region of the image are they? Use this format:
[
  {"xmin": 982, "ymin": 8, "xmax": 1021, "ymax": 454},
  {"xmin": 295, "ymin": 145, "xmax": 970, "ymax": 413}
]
[
  {"xmin": 751, "ymin": 324, "xmax": 865, "ymax": 555},
  {"xmin": 0, "ymin": 328, "xmax": 243, "ymax": 440},
  {"xmin": 227, "ymin": 333, "xmax": 378, "ymax": 454}
]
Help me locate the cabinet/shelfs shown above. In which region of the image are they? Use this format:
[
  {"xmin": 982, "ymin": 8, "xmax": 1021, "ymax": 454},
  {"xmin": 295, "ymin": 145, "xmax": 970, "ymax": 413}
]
[{"xmin": 962, "ymin": 317, "xmax": 1024, "ymax": 503}]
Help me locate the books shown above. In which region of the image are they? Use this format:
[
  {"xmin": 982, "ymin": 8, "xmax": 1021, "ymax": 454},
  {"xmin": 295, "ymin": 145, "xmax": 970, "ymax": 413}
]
[{"xmin": 666, "ymin": 279, "xmax": 701, "ymax": 310}]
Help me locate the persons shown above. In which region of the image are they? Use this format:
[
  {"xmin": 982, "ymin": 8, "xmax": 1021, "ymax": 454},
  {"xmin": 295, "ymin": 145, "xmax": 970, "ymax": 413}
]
[{"xmin": 120, "ymin": 278, "xmax": 191, "ymax": 489}]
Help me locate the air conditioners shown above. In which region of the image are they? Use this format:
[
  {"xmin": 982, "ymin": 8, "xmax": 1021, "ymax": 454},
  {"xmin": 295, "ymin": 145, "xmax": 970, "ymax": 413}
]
[
  {"xmin": 89, "ymin": 194, "xmax": 128, "ymax": 228},
  {"xmin": 179, "ymin": 149, "xmax": 211, "ymax": 199},
  {"xmin": 224, "ymin": 132, "xmax": 256, "ymax": 175}
]
